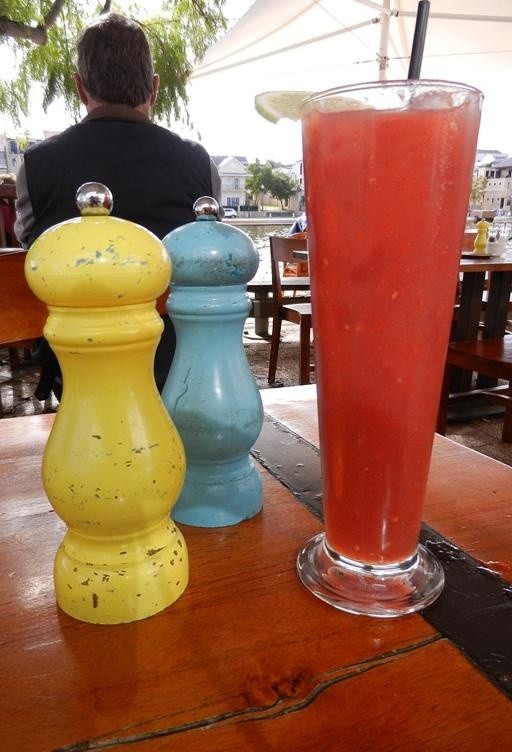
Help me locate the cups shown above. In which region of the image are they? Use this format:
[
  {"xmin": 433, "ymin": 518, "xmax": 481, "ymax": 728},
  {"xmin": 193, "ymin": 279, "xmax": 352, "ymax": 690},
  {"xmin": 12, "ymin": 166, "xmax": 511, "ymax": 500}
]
[{"xmin": 295, "ymin": 80, "xmax": 484, "ymax": 619}]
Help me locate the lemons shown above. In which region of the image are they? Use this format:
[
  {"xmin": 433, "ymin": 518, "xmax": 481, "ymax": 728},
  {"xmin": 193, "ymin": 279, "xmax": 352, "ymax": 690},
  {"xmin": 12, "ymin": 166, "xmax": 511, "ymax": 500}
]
[{"xmin": 254, "ymin": 89, "xmax": 364, "ymax": 124}]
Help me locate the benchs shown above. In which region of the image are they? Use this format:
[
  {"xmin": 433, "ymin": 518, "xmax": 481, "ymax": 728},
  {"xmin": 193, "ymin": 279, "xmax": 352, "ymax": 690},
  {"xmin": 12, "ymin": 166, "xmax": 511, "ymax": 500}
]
[
  {"xmin": 267, "ymin": 235, "xmax": 313, "ymax": 387},
  {"xmin": 439, "ymin": 335, "xmax": 512, "ymax": 445},
  {"xmin": 1, "ymin": 248, "xmax": 174, "ymax": 418}
]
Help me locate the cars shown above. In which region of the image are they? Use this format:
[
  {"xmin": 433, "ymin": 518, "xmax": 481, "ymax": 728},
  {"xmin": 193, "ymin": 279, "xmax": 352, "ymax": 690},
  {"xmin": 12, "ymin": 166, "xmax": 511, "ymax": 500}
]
[{"xmin": 223, "ymin": 208, "xmax": 237, "ymax": 218}]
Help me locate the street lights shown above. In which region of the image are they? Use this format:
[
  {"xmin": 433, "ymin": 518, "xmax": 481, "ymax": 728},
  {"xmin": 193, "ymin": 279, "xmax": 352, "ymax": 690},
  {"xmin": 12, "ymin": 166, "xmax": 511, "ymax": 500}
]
[{"xmin": 260, "ymin": 184, "xmax": 265, "ymax": 211}]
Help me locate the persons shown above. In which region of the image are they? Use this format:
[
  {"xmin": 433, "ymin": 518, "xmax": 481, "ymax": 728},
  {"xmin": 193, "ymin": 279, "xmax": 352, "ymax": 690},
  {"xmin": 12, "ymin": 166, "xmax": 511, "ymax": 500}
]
[
  {"xmin": 13, "ymin": 14, "xmax": 225, "ymax": 403},
  {"xmin": 0, "ymin": 173, "xmax": 16, "ymax": 246},
  {"xmin": 289, "ymin": 210, "xmax": 307, "ymax": 235},
  {"xmin": 184, "ymin": 0, "xmax": 512, "ymax": 79}
]
